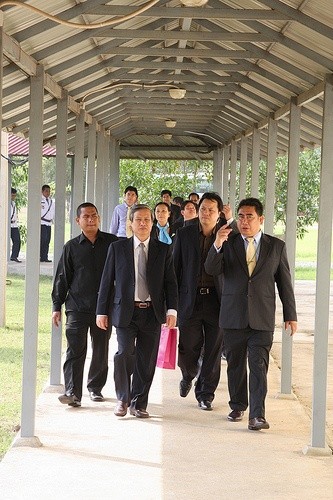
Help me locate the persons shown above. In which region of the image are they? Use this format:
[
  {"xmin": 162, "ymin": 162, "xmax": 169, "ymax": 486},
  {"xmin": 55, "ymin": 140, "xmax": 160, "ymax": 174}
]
[
  {"xmin": 107, "ymin": 186, "xmax": 143, "ymax": 241},
  {"xmin": 147, "ymin": 190, "xmax": 238, "ymax": 361},
  {"xmin": 8, "ymin": 187, "xmax": 22, "ymax": 263},
  {"xmin": 39, "ymin": 184, "xmax": 57, "ymax": 262},
  {"xmin": 94, "ymin": 203, "xmax": 179, "ymax": 418},
  {"xmin": 169, "ymin": 189, "xmax": 227, "ymax": 410},
  {"xmin": 49, "ymin": 203, "xmax": 119, "ymax": 407},
  {"xmin": 203, "ymin": 198, "xmax": 298, "ymax": 431}
]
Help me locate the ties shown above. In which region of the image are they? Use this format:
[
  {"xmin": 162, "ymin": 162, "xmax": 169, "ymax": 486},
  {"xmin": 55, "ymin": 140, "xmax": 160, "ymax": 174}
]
[
  {"xmin": 126, "ymin": 207, "xmax": 133, "ymax": 238},
  {"xmin": 46, "ymin": 198, "xmax": 49, "ymax": 205},
  {"xmin": 245, "ymin": 238, "xmax": 258, "ymax": 277},
  {"xmin": 138, "ymin": 243, "xmax": 149, "ymax": 301}
]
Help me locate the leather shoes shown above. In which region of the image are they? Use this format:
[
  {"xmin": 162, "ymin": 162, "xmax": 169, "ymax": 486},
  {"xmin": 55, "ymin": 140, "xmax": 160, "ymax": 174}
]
[
  {"xmin": 248, "ymin": 417, "xmax": 270, "ymax": 430},
  {"xmin": 228, "ymin": 410, "xmax": 244, "ymax": 421},
  {"xmin": 58, "ymin": 392, "xmax": 82, "ymax": 406},
  {"xmin": 114, "ymin": 400, "xmax": 130, "ymax": 417},
  {"xmin": 89, "ymin": 392, "xmax": 104, "ymax": 401},
  {"xmin": 198, "ymin": 401, "xmax": 213, "ymax": 411},
  {"xmin": 179, "ymin": 379, "xmax": 192, "ymax": 397},
  {"xmin": 129, "ymin": 406, "xmax": 149, "ymax": 418}
]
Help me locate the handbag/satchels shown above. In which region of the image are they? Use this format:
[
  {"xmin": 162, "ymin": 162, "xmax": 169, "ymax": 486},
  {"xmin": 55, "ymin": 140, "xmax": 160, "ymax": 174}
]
[{"xmin": 155, "ymin": 323, "xmax": 177, "ymax": 370}]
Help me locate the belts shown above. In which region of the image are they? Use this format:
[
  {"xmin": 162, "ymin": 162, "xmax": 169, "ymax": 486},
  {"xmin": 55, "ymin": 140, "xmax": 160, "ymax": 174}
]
[
  {"xmin": 198, "ymin": 287, "xmax": 216, "ymax": 294},
  {"xmin": 11, "ymin": 221, "xmax": 19, "ymax": 223},
  {"xmin": 135, "ymin": 302, "xmax": 152, "ymax": 309},
  {"xmin": 41, "ymin": 217, "xmax": 53, "ymax": 222}
]
